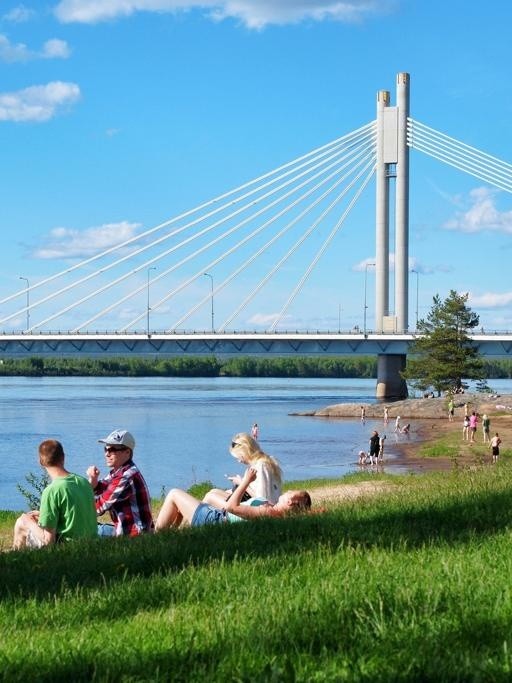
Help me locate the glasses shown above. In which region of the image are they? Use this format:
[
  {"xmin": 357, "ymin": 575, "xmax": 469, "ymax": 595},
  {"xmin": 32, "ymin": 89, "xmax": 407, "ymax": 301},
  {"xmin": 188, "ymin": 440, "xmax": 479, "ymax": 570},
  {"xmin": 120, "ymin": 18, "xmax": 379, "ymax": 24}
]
[
  {"xmin": 231, "ymin": 440, "xmax": 242, "ymax": 448},
  {"xmin": 104, "ymin": 447, "xmax": 128, "ymax": 452}
]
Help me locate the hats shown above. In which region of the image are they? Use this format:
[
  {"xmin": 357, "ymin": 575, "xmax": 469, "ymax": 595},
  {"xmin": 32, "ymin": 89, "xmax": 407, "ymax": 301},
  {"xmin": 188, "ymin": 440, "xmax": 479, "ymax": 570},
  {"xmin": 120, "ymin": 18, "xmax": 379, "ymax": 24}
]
[{"xmin": 98, "ymin": 428, "xmax": 135, "ymax": 451}]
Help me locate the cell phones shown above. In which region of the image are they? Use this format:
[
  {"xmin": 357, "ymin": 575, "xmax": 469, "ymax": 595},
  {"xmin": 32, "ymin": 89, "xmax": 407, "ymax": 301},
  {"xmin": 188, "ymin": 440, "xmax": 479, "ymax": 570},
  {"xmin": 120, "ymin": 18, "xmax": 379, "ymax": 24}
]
[{"xmin": 224, "ymin": 474, "xmax": 236, "ymax": 479}]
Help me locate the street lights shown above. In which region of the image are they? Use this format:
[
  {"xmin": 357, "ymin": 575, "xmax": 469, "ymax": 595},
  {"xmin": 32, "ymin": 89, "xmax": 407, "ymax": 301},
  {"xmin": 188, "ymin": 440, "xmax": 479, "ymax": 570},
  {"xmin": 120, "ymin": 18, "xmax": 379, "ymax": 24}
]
[
  {"xmin": 363, "ymin": 263, "xmax": 375, "ymax": 333},
  {"xmin": 411, "ymin": 269, "xmax": 419, "ymax": 329},
  {"xmin": 146, "ymin": 266, "xmax": 156, "ymax": 332},
  {"xmin": 19, "ymin": 276, "xmax": 30, "ymax": 330},
  {"xmin": 203, "ymin": 272, "xmax": 215, "ymax": 331}
]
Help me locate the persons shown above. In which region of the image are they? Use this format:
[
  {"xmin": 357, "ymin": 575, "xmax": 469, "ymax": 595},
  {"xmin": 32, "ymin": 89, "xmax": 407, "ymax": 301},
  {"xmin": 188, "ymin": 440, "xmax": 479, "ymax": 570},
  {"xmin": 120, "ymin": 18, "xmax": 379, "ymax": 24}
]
[
  {"xmin": 152, "ymin": 465, "xmax": 313, "ymax": 534},
  {"xmin": 86, "ymin": 427, "xmax": 157, "ymax": 537},
  {"xmin": 205, "ymin": 431, "xmax": 285, "ymax": 503},
  {"xmin": 354, "ymin": 385, "xmax": 502, "ymax": 470},
  {"xmin": 252, "ymin": 423, "xmax": 258, "ymax": 439},
  {"xmin": 10, "ymin": 439, "xmax": 99, "ymax": 554}
]
